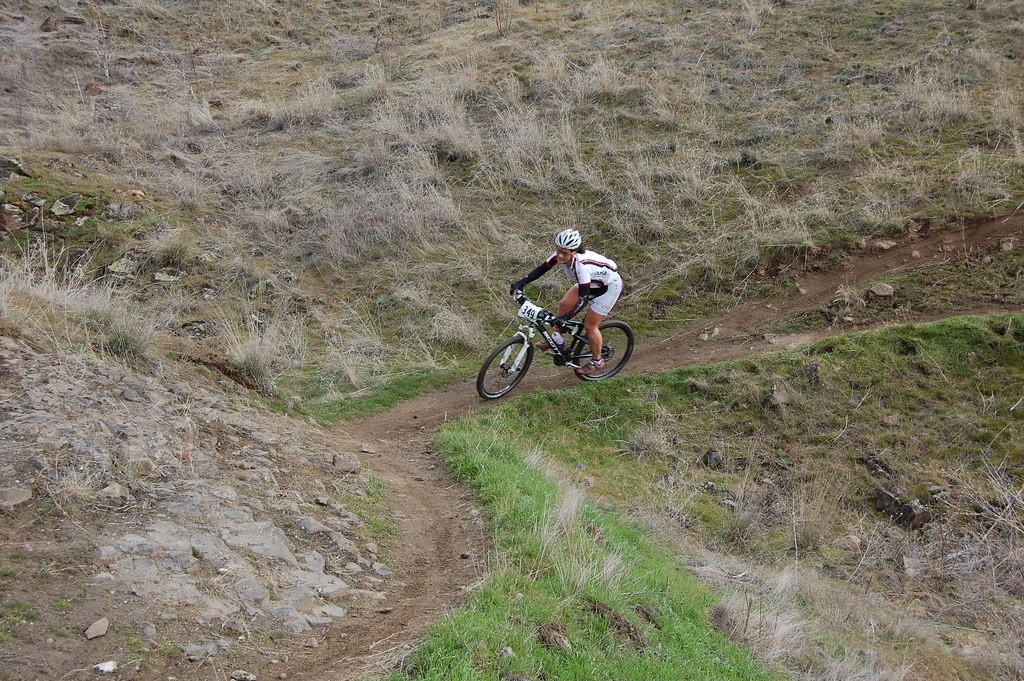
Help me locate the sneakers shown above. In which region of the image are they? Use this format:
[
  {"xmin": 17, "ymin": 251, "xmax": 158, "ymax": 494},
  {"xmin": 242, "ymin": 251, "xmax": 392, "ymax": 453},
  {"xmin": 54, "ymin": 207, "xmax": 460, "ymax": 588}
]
[
  {"xmin": 535, "ymin": 339, "xmax": 565, "ymax": 350},
  {"xmin": 576, "ymin": 359, "xmax": 603, "ymax": 375}
]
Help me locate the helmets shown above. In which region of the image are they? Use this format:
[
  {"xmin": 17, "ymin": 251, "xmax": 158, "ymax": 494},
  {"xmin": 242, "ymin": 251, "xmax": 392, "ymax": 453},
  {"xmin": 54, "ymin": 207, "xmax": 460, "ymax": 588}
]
[{"xmin": 555, "ymin": 229, "xmax": 581, "ymax": 250}]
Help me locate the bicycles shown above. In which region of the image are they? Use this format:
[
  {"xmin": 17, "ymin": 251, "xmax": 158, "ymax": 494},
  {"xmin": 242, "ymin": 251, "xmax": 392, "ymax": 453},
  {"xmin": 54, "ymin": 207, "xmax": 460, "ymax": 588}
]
[{"xmin": 476, "ymin": 288, "xmax": 635, "ymax": 400}]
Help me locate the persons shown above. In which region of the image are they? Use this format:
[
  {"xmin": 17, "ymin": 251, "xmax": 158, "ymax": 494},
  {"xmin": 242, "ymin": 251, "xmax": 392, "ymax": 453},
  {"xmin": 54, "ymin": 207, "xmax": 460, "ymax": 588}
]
[{"xmin": 511, "ymin": 229, "xmax": 622, "ymax": 375}]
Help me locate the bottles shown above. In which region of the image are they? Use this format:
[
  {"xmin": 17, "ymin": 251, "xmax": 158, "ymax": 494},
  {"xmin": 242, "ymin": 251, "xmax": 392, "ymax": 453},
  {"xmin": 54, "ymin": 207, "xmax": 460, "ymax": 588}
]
[{"xmin": 551, "ymin": 331, "xmax": 568, "ymax": 351}]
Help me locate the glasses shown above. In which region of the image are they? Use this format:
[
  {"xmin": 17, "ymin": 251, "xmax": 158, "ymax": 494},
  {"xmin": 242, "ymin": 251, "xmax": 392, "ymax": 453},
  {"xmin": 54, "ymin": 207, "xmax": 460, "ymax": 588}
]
[{"xmin": 556, "ymin": 247, "xmax": 578, "ymax": 255}]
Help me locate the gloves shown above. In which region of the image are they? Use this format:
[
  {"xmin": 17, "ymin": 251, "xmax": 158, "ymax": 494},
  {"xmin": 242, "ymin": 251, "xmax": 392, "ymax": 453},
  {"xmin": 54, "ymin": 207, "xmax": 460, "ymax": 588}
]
[
  {"xmin": 509, "ymin": 279, "xmax": 525, "ymax": 294},
  {"xmin": 550, "ymin": 314, "xmax": 571, "ymax": 329}
]
